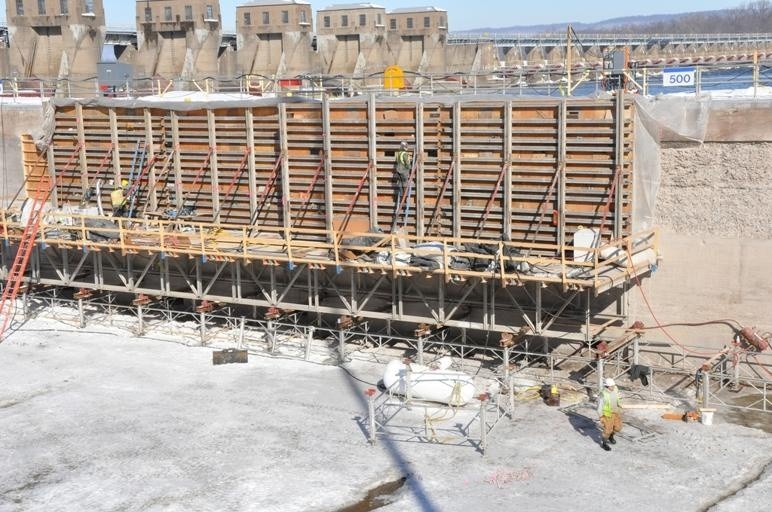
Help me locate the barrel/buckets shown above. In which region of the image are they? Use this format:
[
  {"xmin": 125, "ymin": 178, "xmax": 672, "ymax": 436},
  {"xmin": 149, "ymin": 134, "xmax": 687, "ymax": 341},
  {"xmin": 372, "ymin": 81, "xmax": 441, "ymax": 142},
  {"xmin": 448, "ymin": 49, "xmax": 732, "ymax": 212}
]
[
  {"xmin": 702, "ymin": 412, "xmax": 713, "ymax": 425},
  {"xmin": 574, "ymin": 229, "xmax": 600, "ymax": 263}
]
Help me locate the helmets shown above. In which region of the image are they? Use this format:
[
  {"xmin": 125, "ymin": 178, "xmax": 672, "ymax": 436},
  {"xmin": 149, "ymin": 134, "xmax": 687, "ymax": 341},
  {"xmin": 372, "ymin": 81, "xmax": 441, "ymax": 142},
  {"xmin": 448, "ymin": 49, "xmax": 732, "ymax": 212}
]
[{"xmin": 603, "ymin": 378, "xmax": 616, "ymax": 387}]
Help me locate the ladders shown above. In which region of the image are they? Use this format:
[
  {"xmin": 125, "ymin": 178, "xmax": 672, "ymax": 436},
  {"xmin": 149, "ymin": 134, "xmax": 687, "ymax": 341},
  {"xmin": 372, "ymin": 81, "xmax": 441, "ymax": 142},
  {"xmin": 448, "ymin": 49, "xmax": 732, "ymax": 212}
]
[
  {"xmin": 0, "ymin": 173, "xmax": 51, "ymax": 342},
  {"xmin": 393, "ymin": 149, "xmax": 417, "ymax": 233},
  {"xmin": 122, "ymin": 139, "xmax": 148, "ymax": 217}
]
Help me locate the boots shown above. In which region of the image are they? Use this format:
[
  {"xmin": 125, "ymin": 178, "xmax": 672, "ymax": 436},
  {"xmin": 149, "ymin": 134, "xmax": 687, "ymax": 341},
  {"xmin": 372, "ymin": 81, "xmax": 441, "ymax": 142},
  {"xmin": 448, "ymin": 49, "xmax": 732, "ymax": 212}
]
[
  {"xmin": 608, "ymin": 433, "xmax": 616, "ymax": 444},
  {"xmin": 602, "ymin": 441, "xmax": 611, "ymax": 451}
]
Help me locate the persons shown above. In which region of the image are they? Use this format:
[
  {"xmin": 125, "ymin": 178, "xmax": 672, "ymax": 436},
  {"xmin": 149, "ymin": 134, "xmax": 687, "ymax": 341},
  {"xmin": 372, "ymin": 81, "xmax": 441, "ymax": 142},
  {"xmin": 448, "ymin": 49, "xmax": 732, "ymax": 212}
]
[
  {"xmin": 391, "ymin": 140, "xmax": 413, "ymax": 198},
  {"xmin": 107, "ymin": 184, "xmax": 129, "ymax": 224},
  {"xmin": 592, "ymin": 377, "xmax": 624, "ymax": 453}
]
